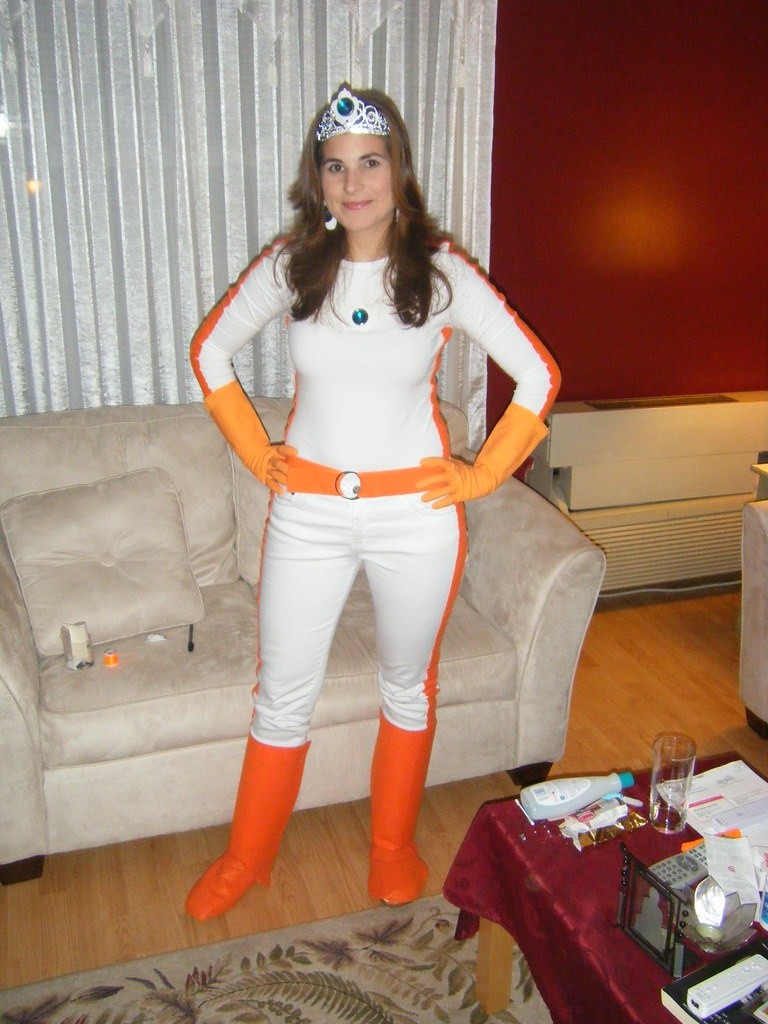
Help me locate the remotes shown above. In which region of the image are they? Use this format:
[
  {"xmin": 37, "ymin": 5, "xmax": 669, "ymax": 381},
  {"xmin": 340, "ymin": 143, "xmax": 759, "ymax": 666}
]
[
  {"xmin": 648, "ymin": 836, "xmax": 735, "ymax": 892},
  {"xmin": 686, "ymin": 954, "xmax": 768, "ymax": 1020}
]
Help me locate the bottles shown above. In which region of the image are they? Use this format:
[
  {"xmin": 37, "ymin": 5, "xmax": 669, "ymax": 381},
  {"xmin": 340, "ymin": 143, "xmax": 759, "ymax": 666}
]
[{"xmin": 519, "ymin": 771, "xmax": 635, "ymax": 820}]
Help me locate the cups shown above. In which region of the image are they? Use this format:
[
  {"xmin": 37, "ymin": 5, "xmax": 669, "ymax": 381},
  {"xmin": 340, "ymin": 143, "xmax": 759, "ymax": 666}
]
[{"xmin": 647, "ymin": 734, "xmax": 698, "ymax": 834}]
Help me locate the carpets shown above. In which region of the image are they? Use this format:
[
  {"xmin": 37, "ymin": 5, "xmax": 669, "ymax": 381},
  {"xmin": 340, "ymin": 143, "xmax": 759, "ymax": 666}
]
[{"xmin": 0, "ymin": 895, "xmax": 552, "ymax": 1024}]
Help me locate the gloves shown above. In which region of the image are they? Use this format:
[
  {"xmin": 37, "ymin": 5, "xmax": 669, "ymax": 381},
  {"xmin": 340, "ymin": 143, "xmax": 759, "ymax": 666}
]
[
  {"xmin": 418, "ymin": 402, "xmax": 550, "ymax": 509},
  {"xmin": 203, "ymin": 379, "xmax": 298, "ymax": 495}
]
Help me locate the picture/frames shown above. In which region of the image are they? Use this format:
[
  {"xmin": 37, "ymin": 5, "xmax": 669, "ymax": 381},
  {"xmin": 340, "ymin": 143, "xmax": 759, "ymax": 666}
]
[{"xmin": 616, "ymin": 842, "xmax": 693, "ymax": 978}]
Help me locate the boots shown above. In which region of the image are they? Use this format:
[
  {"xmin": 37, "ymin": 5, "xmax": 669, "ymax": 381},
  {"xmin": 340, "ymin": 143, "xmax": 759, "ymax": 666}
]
[
  {"xmin": 186, "ymin": 732, "xmax": 312, "ymax": 922},
  {"xmin": 368, "ymin": 707, "xmax": 437, "ymax": 908}
]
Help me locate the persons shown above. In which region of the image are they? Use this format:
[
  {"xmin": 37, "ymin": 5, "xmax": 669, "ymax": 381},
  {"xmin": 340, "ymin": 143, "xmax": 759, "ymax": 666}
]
[{"xmin": 185, "ymin": 84, "xmax": 564, "ymax": 922}]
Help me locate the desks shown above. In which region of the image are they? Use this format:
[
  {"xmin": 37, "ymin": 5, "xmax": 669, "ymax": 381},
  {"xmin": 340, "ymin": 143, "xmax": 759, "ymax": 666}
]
[{"xmin": 443, "ymin": 753, "xmax": 768, "ymax": 1024}]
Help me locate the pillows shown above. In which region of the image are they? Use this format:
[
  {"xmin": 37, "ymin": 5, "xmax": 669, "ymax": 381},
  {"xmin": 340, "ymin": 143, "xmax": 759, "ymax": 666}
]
[
  {"xmin": 228, "ymin": 443, "xmax": 269, "ymax": 583},
  {"xmin": 0, "ymin": 467, "xmax": 205, "ymax": 657}
]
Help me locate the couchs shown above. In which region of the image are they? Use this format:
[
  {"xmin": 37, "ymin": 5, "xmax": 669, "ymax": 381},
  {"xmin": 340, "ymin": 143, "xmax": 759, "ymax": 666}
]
[{"xmin": 1, "ymin": 394, "xmax": 605, "ymax": 885}]
